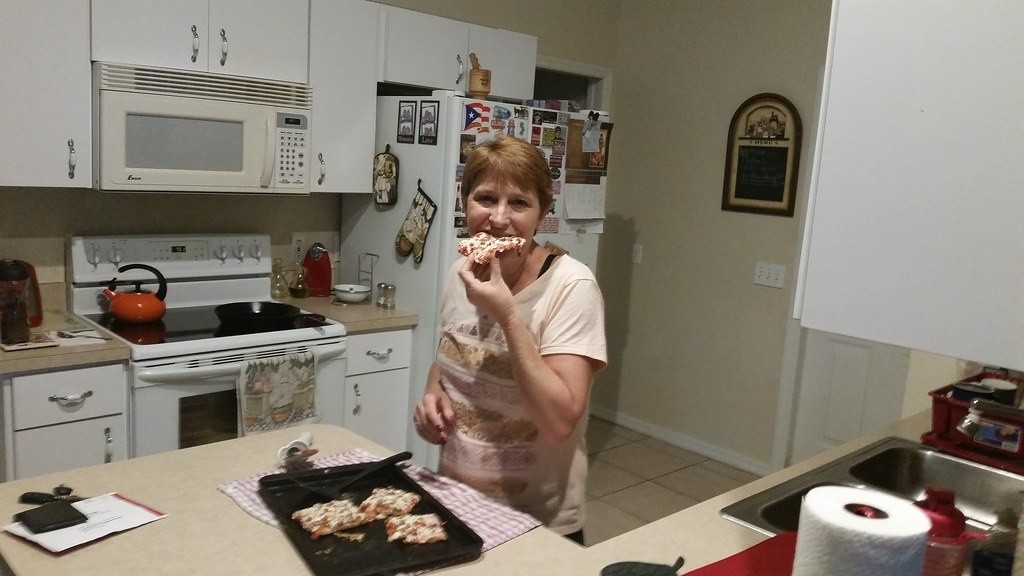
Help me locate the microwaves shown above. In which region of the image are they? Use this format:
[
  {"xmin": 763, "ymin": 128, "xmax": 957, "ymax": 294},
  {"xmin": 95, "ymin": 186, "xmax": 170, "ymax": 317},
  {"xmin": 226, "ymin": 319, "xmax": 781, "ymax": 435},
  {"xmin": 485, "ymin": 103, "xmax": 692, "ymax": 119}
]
[{"xmin": 92, "ymin": 60, "xmax": 314, "ymax": 197}]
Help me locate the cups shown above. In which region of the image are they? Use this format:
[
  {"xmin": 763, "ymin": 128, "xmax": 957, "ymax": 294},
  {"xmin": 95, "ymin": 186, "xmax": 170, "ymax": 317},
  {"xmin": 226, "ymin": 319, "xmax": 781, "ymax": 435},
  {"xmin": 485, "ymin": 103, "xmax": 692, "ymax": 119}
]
[
  {"xmin": 953, "ymin": 382, "xmax": 994, "ymax": 402},
  {"xmin": 980, "ymin": 377, "xmax": 1018, "ymax": 407}
]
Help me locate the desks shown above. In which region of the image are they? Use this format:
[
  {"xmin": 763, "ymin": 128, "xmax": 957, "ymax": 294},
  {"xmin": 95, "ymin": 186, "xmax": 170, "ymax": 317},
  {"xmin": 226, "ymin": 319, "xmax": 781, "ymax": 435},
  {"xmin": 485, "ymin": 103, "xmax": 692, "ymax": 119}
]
[{"xmin": 1, "ymin": 421, "xmax": 589, "ymax": 574}]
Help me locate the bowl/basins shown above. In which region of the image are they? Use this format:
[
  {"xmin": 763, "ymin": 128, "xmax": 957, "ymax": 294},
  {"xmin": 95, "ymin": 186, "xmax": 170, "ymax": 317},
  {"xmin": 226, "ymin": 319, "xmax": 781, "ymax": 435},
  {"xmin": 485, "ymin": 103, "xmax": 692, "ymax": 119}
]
[{"xmin": 333, "ymin": 283, "xmax": 372, "ymax": 302}]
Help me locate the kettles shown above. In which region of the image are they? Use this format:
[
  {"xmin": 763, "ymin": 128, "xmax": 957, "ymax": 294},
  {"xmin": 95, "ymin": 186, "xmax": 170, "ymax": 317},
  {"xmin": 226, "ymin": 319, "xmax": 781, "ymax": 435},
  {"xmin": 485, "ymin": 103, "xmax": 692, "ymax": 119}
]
[{"xmin": 101, "ymin": 263, "xmax": 168, "ymax": 324}]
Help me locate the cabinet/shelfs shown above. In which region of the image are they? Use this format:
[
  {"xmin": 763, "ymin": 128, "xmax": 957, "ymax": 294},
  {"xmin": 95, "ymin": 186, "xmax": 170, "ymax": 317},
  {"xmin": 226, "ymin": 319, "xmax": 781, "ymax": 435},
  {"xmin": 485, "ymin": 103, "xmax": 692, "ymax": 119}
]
[
  {"xmin": 1, "ymin": 0, "xmax": 95, "ymax": 189},
  {"xmin": 794, "ymin": 0, "xmax": 1024, "ymax": 374},
  {"xmin": 305, "ymin": 0, "xmax": 537, "ymax": 194},
  {"xmin": 0, "ymin": 362, "xmax": 130, "ymax": 481},
  {"xmin": 345, "ymin": 327, "xmax": 412, "ymax": 456}
]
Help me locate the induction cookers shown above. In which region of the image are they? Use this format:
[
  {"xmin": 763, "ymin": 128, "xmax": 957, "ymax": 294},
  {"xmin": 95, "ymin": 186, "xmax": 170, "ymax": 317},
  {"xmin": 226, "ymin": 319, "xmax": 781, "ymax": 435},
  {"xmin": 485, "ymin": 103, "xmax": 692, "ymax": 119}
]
[{"xmin": 65, "ymin": 232, "xmax": 347, "ymax": 361}]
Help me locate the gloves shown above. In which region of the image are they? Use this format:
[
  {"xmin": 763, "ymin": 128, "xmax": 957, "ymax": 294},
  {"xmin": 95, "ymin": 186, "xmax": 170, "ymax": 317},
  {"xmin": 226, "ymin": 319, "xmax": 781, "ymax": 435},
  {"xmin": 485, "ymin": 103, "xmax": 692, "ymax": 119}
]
[{"xmin": 395, "ymin": 188, "xmax": 437, "ymax": 263}]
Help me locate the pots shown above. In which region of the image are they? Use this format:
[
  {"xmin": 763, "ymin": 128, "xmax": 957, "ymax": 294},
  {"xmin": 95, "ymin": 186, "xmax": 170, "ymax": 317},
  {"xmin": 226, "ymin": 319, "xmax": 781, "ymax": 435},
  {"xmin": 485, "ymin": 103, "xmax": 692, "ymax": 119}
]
[{"xmin": 215, "ymin": 302, "xmax": 326, "ymax": 325}]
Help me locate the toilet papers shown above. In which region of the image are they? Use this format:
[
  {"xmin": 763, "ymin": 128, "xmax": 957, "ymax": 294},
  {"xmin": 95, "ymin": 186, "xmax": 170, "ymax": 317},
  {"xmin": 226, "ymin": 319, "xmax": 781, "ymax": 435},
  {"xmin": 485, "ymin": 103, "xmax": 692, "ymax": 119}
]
[{"xmin": 791, "ymin": 485, "xmax": 933, "ymax": 576}]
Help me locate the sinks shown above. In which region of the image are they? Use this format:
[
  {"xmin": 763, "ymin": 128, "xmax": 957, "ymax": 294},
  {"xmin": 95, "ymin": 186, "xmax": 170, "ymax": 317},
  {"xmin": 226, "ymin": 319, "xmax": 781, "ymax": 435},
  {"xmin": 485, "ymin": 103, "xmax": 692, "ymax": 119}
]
[
  {"xmin": 792, "ymin": 436, "xmax": 1024, "ymax": 532},
  {"xmin": 725, "ymin": 480, "xmax": 993, "ymax": 532}
]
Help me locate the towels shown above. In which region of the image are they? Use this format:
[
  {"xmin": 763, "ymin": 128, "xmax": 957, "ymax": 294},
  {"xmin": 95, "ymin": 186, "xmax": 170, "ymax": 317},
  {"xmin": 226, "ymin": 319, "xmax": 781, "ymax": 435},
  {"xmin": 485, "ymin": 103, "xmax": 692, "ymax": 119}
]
[{"xmin": 235, "ymin": 350, "xmax": 322, "ymax": 438}]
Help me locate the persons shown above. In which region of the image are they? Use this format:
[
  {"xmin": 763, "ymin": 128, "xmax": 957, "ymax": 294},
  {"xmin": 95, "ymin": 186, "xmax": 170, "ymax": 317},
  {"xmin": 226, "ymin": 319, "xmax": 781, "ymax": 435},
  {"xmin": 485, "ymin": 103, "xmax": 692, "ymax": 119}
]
[
  {"xmin": 412, "ymin": 134, "xmax": 609, "ymax": 548},
  {"xmin": 554, "ymin": 126, "xmax": 565, "ymax": 141}
]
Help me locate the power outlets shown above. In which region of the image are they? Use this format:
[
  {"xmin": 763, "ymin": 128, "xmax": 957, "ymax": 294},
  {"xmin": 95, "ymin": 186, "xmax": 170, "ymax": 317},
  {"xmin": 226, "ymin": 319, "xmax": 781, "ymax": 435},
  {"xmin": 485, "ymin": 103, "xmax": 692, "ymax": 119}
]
[
  {"xmin": 631, "ymin": 243, "xmax": 642, "ymax": 263},
  {"xmin": 752, "ymin": 262, "xmax": 788, "ymax": 289}
]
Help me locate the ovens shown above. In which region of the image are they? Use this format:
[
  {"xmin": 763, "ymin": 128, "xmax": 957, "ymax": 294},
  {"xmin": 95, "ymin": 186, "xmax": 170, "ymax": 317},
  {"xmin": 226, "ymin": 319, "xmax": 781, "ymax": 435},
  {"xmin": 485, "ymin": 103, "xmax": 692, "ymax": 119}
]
[{"xmin": 130, "ymin": 336, "xmax": 347, "ymax": 458}]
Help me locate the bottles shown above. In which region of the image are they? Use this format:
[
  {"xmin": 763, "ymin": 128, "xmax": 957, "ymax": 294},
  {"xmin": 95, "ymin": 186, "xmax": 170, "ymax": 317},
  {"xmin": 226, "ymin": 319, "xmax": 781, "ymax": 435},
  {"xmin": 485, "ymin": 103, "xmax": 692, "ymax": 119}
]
[
  {"xmin": 383, "ymin": 285, "xmax": 396, "ymax": 310},
  {"xmin": 271, "ymin": 258, "xmax": 288, "ymax": 298},
  {"xmin": 288, "ymin": 260, "xmax": 310, "ymax": 298},
  {"xmin": 911, "ymin": 484, "xmax": 968, "ymax": 576},
  {"xmin": 375, "ymin": 282, "xmax": 387, "ymax": 307},
  {"xmin": 971, "ymin": 507, "xmax": 1018, "ymax": 576}
]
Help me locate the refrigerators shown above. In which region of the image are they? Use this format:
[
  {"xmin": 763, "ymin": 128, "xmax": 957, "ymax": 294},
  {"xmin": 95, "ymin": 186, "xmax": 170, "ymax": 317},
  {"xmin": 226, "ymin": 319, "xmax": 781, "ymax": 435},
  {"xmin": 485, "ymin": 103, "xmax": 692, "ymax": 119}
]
[{"xmin": 339, "ymin": 91, "xmax": 611, "ymax": 473}]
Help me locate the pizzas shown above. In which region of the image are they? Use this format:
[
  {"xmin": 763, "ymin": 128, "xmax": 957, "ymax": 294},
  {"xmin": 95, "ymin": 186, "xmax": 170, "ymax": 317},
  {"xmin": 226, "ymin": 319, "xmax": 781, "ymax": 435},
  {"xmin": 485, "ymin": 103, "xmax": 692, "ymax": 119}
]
[
  {"xmin": 357, "ymin": 487, "xmax": 420, "ymax": 518},
  {"xmin": 290, "ymin": 499, "xmax": 371, "ymax": 540},
  {"xmin": 457, "ymin": 231, "xmax": 526, "ymax": 265},
  {"xmin": 384, "ymin": 512, "xmax": 447, "ymax": 543}
]
